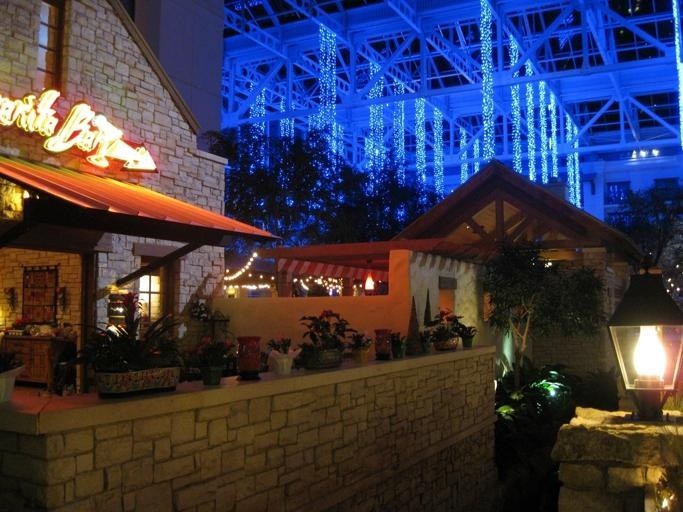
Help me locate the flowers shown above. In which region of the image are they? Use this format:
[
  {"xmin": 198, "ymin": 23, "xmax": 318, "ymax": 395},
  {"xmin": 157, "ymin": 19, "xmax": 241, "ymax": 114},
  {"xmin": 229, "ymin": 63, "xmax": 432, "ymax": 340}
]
[
  {"xmin": 348, "ymin": 331, "xmax": 373, "ymax": 350},
  {"xmin": 80, "ymin": 292, "xmax": 191, "ymax": 370},
  {"xmin": 425, "ymin": 305, "xmax": 465, "ymax": 339},
  {"xmin": 300, "ymin": 309, "xmax": 358, "ymax": 348},
  {"xmin": 193, "ymin": 337, "xmax": 233, "ymax": 369}
]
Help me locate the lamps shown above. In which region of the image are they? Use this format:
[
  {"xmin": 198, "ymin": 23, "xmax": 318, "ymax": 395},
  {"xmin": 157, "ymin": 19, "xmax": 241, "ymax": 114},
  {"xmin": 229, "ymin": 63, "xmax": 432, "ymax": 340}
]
[{"xmin": 606, "ymin": 252, "xmax": 683, "ymax": 423}]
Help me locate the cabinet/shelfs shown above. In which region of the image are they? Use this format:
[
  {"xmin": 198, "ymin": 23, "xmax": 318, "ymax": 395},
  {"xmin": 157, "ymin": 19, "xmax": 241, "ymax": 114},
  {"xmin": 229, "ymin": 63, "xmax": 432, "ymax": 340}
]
[{"xmin": 1, "ymin": 338, "xmax": 52, "ymax": 391}]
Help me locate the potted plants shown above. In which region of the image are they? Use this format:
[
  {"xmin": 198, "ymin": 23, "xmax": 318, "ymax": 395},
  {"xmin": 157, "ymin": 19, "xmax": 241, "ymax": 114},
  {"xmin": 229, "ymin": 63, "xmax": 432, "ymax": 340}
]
[
  {"xmin": 266, "ymin": 335, "xmax": 295, "ymax": 376},
  {"xmin": 390, "ymin": 332, "xmax": 413, "ymax": 359},
  {"xmin": 459, "ymin": 326, "xmax": 480, "ymax": 348}
]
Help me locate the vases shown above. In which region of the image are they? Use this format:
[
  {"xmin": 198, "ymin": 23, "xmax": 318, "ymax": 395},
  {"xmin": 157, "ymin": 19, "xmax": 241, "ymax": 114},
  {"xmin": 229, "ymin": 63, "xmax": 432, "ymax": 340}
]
[
  {"xmin": 236, "ymin": 335, "xmax": 262, "ymax": 383},
  {"xmin": 202, "ymin": 367, "xmax": 220, "ymax": 386},
  {"xmin": 375, "ymin": 329, "xmax": 392, "ymax": 362},
  {"xmin": 352, "ymin": 347, "xmax": 370, "ymax": 364},
  {"xmin": 95, "ymin": 366, "xmax": 181, "ymax": 396},
  {"xmin": 301, "ymin": 347, "xmax": 345, "ymax": 370},
  {"xmin": 432, "ymin": 336, "xmax": 459, "ymax": 350}
]
